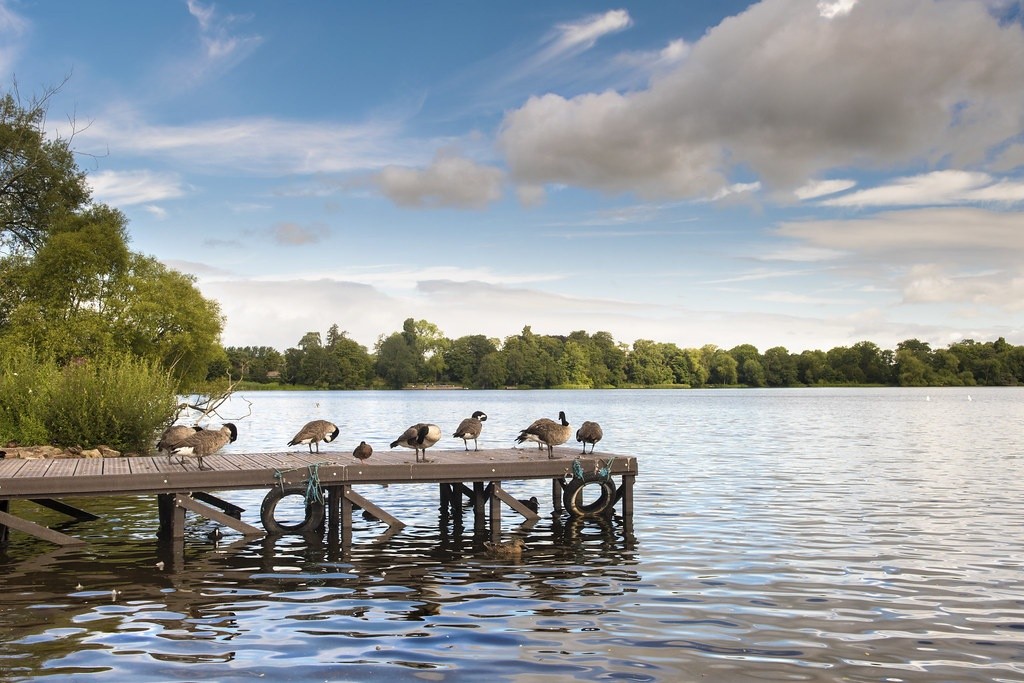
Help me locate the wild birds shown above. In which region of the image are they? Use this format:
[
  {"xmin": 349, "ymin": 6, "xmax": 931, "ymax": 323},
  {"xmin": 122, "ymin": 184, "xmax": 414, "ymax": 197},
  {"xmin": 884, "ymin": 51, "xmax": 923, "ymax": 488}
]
[
  {"xmin": 155, "ymin": 423, "xmax": 237, "ymax": 470},
  {"xmin": 353, "ymin": 441, "xmax": 372, "ymax": 464},
  {"xmin": 390, "ymin": 423, "xmax": 441, "ymax": 464},
  {"xmin": 514, "ymin": 412, "xmax": 573, "ymax": 459},
  {"xmin": 208, "ymin": 527, "xmax": 222, "ymax": 541},
  {"xmin": 510, "ymin": 497, "xmax": 540, "ymax": 514},
  {"xmin": 452, "ymin": 411, "xmax": 487, "ymax": 451},
  {"xmin": 576, "ymin": 421, "xmax": 603, "ymax": 455},
  {"xmin": 287, "ymin": 420, "xmax": 339, "ymax": 454},
  {"xmin": 483, "ymin": 538, "xmax": 529, "ymax": 554}
]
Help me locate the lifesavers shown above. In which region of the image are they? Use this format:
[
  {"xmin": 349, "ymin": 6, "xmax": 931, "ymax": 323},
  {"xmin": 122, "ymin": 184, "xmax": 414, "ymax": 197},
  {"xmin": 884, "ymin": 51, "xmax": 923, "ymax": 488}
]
[
  {"xmin": 563, "ymin": 472, "xmax": 616, "ymax": 516},
  {"xmin": 261, "ymin": 482, "xmax": 324, "ymax": 536}
]
